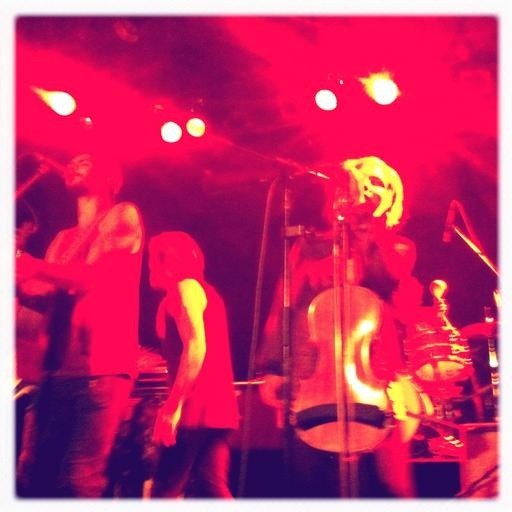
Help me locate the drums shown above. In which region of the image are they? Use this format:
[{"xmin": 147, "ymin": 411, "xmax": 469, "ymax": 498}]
[
  {"xmin": 399, "ymin": 375, "xmax": 434, "ymax": 440},
  {"xmin": 406, "ymin": 330, "xmax": 469, "ymax": 381}
]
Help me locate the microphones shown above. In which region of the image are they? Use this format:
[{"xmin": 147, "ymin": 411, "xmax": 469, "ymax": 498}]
[
  {"xmin": 442, "ymin": 198, "xmax": 460, "ymax": 247},
  {"xmin": 270, "ymin": 157, "xmax": 331, "ymax": 186},
  {"xmin": 34, "ymin": 152, "xmax": 75, "ymax": 182}
]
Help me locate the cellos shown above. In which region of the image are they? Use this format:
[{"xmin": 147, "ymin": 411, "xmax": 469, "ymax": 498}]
[{"xmin": 295, "ymin": 174, "xmax": 392, "ymax": 451}]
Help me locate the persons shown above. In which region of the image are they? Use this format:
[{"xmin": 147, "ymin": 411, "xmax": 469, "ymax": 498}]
[
  {"xmin": 17, "ymin": 142, "xmax": 148, "ymax": 497},
  {"xmin": 247, "ymin": 181, "xmax": 427, "ymax": 498},
  {"xmin": 142, "ymin": 231, "xmax": 240, "ymax": 497}
]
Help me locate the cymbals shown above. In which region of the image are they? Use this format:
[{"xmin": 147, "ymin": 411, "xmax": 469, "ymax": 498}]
[{"xmin": 465, "ymin": 323, "xmax": 499, "ymax": 340}]
